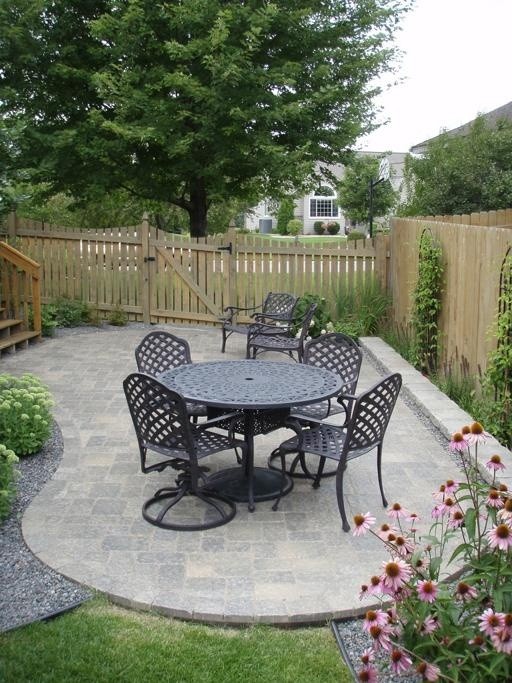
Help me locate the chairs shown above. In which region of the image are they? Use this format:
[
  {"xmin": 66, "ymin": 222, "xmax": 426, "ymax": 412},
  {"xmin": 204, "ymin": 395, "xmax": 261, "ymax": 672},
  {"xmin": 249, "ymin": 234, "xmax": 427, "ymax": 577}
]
[
  {"xmin": 122, "ymin": 369, "xmax": 245, "ymax": 532},
  {"xmin": 271, "ymin": 372, "xmax": 404, "ymax": 533},
  {"xmin": 134, "ymin": 332, "xmax": 242, "ymax": 465},
  {"xmin": 220, "ymin": 291, "xmax": 318, "ymax": 363},
  {"xmin": 266, "ymin": 331, "xmax": 362, "ymax": 479}
]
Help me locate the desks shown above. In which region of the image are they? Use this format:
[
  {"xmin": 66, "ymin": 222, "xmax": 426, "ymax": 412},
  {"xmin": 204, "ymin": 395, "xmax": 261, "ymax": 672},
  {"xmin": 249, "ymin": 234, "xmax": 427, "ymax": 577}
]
[{"xmin": 151, "ymin": 359, "xmax": 344, "ymax": 506}]
